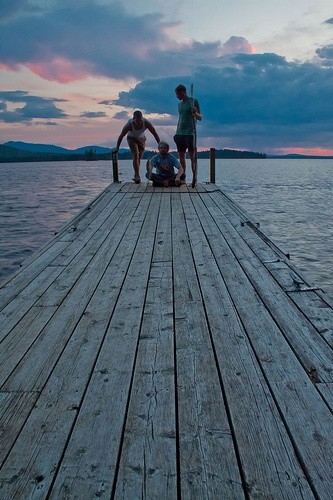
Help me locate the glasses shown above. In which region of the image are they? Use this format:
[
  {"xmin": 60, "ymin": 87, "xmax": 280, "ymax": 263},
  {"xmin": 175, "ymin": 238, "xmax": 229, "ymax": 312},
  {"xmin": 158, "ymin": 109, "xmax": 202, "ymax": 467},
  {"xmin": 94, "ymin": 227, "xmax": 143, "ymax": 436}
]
[{"xmin": 159, "ymin": 146, "xmax": 168, "ymax": 149}]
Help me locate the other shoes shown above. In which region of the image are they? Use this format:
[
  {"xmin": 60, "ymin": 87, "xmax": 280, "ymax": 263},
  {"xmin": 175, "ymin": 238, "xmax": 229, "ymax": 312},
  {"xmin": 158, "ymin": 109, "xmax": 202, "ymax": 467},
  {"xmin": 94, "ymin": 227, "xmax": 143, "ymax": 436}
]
[
  {"xmin": 175, "ymin": 172, "xmax": 186, "ymax": 180},
  {"xmin": 132, "ymin": 176, "xmax": 140, "ymax": 184},
  {"xmin": 192, "ymin": 180, "xmax": 198, "ymax": 187},
  {"xmin": 163, "ymin": 179, "xmax": 173, "ymax": 186}
]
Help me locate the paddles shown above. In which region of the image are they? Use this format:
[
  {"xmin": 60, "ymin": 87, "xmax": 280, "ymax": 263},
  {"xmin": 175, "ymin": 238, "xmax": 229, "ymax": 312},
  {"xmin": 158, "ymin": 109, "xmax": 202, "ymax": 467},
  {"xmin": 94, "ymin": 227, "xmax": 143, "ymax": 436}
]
[{"xmin": 190, "ymin": 83, "xmax": 198, "ymax": 189}]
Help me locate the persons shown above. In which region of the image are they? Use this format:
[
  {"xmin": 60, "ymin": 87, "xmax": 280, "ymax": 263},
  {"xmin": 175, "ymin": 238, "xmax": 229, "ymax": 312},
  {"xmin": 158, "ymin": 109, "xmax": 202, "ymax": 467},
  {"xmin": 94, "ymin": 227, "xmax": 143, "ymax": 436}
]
[
  {"xmin": 171, "ymin": 86, "xmax": 201, "ymax": 186},
  {"xmin": 146, "ymin": 139, "xmax": 186, "ymax": 188},
  {"xmin": 112, "ymin": 109, "xmax": 160, "ymax": 184}
]
